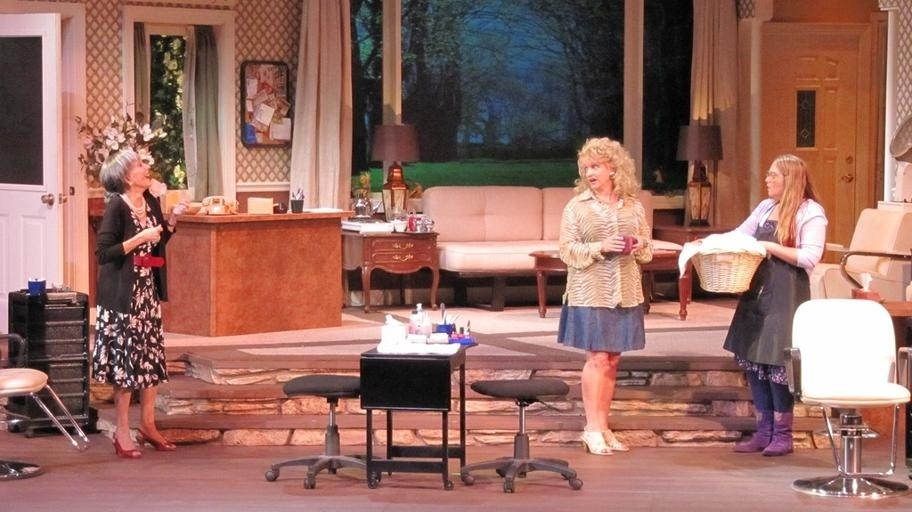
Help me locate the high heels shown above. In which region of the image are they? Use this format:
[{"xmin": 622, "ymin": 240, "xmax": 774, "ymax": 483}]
[
  {"xmin": 136, "ymin": 429, "xmax": 177, "ymax": 453},
  {"xmin": 112, "ymin": 431, "xmax": 141, "ymax": 459},
  {"xmin": 581, "ymin": 429, "xmax": 630, "ymax": 454}
]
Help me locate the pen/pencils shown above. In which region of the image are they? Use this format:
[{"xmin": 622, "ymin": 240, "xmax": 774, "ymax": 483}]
[{"xmin": 292, "ymin": 187, "xmax": 304, "ymax": 201}]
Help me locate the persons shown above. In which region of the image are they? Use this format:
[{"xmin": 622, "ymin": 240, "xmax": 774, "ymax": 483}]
[
  {"xmin": 89, "ymin": 146, "xmax": 191, "ymax": 459},
  {"xmin": 557, "ymin": 135, "xmax": 655, "ymax": 456},
  {"xmin": 688, "ymin": 153, "xmax": 828, "ymax": 458}
]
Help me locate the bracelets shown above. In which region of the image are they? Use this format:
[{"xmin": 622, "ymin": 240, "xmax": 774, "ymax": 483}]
[{"xmin": 167, "ymin": 220, "xmax": 178, "ymax": 229}]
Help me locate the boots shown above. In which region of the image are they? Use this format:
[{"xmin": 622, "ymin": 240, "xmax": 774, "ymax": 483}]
[{"xmin": 733, "ymin": 410, "xmax": 793, "ymax": 455}]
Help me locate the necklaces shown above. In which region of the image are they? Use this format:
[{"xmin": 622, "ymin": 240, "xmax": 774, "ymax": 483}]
[{"xmin": 120, "ymin": 191, "xmax": 147, "ymax": 219}]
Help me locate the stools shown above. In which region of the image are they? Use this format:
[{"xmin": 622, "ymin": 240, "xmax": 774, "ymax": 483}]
[
  {"xmin": 265, "ymin": 372, "xmax": 384, "ymax": 489},
  {"xmin": 1, "ymin": 367, "xmax": 91, "ymax": 452},
  {"xmin": 459, "ymin": 378, "xmax": 583, "ymax": 493}
]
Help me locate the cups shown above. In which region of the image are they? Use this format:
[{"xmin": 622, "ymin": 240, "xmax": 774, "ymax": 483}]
[
  {"xmin": 27, "ymin": 278, "xmax": 48, "ymax": 298},
  {"xmin": 291, "ymin": 199, "xmax": 304, "ymax": 212},
  {"xmin": 434, "ymin": 323, "xmax": 455, "ymax": 338},
  {"xmin": 621, "ymin": 235, "xmax": 638, "ymax": 254},
  {"xmin": 394, "ymin": 220, "xmax": 407, "ymax": 232}
]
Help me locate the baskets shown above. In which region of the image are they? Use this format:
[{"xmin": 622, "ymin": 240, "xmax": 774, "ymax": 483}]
[{"xmin": 690, "ymin": 251, "xmax": 765, "ymax": 295}]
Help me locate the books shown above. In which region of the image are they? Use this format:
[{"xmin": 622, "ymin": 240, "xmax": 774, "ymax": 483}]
[
  {"xmin": 43, "ymin": 291, "xmax": 77, "ymax": 309},
  {"xmin": 340, "ymin": 221, "xmax": 395, "ymax": 233}
]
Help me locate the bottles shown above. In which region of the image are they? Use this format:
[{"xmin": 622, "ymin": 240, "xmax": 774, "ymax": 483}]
[
  {"xmin": 408, "ymin": 211, "xmax": 432, "ymax": 232},
  {"xmin": 408, "ymin": 302, "xmax": 433, "ymax": 338}
]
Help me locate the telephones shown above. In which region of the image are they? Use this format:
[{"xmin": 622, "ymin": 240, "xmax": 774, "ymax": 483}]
[{"xmin": 202, "ymin": 196, "xmax": 232, "ymax": 214}]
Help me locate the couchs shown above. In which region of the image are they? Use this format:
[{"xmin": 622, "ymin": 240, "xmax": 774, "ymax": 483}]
[
  {"xmin": 806, "ymin": 205, "xmax": 912, "ymax": 315},
  {"xmin": 423, "ymin": 184, "xmax": 681, "ymax": 312}
]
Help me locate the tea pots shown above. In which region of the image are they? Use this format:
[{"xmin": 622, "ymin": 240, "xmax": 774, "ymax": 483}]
[{"xmin": 355, "ymin": 189, "xmax": 382, "ymax": 218}]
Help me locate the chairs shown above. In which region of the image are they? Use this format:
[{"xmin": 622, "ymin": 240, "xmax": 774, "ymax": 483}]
[{"xmin": 782, "ymin": 297, "xmax": 910, "ymax": 498}]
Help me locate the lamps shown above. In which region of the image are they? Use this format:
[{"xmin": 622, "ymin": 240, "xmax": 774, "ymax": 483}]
[
  {"xmin": 366, "ymin": 125, "xmax": 426, "ymax": 223},
  {"xmin": 676, "ymin": 116, "xmax": 725, "ymax": 227}
]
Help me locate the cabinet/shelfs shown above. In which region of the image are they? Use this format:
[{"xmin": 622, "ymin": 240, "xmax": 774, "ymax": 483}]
[{"xmin": 8, "ymin": 290, "xmax": 89, "ymax": 439}]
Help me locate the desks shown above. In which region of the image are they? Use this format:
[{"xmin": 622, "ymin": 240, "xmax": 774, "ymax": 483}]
[
  {"xmin": 652, "ymin": 225, "xmax": 737, "ymax": 304},
  {"xmin": 342, "ymin": 217, "xmax": 443, "ymax": 315},
  {"xmin": 90, "ymin": 194, "xmax": 357, "ymax": 336},
  {"xmin": 360, "ymin": 339, "xmax": 479, "ymax": 492}
]
